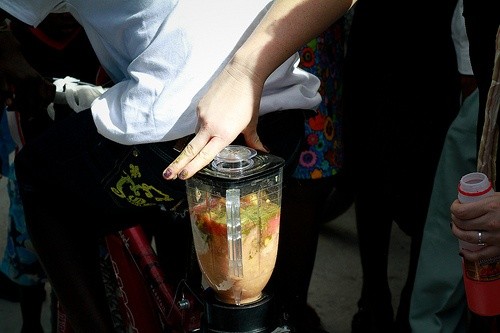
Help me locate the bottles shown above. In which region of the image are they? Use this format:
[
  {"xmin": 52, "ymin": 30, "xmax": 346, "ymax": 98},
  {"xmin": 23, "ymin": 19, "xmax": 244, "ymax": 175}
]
[{"xmin": 457, "ymin": 172, "xmax": 500, "ymax": 315}]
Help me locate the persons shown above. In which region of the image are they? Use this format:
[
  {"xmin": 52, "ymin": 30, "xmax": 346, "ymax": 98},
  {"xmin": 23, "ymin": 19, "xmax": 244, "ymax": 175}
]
[{"xmin": 0, "ymin": 0, "xmax": 500, "ymax": 333}]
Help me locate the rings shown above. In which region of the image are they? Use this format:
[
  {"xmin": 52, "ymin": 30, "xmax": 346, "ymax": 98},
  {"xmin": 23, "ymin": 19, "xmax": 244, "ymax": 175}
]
[{"xmin": 478, "ymin": 231, "xmax": 483, "ymax": 244}]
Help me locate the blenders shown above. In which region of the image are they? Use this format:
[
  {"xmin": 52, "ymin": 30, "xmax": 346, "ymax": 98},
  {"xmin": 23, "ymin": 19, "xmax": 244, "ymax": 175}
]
[{"xmin": 179, "ymin": 146, "xmax": 286, "ymax": 333}]
